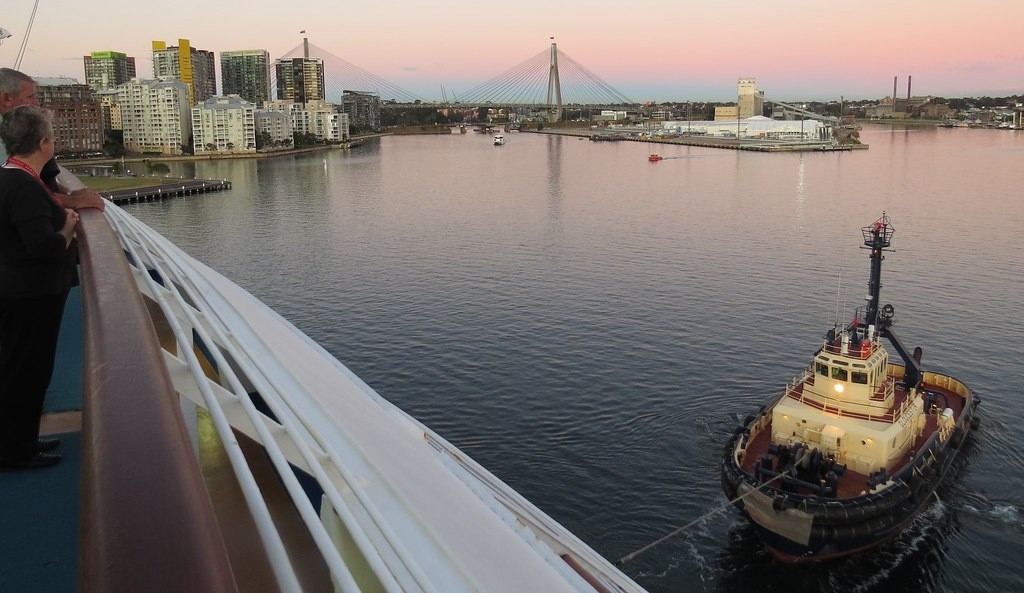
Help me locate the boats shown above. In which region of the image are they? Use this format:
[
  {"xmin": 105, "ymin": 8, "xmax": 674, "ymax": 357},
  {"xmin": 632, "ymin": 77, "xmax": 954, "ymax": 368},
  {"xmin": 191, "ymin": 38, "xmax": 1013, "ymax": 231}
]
[
  {"xmin": 720, "ymin": 209, "xmax": 981, "ymax": 567},
  {"xmin": 493, "ymin": 135, "xmax": 506, "ymax": 146},
  {"xmin": 648, "ymin": 152, "xmax": 663, "ymax": 162}
]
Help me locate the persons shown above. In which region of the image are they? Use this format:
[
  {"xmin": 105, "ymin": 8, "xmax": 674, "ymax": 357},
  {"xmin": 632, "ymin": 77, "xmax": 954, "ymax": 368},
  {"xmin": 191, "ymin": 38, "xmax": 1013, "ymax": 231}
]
[
  {"xmin": 0, "ymin": 102, "xmax": 80, "ymax": 473},
  {"xmin": 1, "ymin": 66, "xmax": 106, "ymax": 214}
]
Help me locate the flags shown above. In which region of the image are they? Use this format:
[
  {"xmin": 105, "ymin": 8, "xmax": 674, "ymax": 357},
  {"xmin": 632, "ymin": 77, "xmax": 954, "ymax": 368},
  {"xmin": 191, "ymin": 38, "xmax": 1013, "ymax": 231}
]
[
  {"xmin": 300, "ymin": 30, "xmax": 305, "ymax": 33},
  {"xmin": 550, "ymin": 36, "xmax": 554, "ymax": 39}
]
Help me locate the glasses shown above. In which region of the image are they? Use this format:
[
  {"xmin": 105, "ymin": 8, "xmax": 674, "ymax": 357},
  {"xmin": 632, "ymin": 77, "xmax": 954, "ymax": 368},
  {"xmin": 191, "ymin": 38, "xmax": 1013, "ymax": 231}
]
[{"xmin": 47, "ymin": 136, "xmax": 59, "ymax": 147}]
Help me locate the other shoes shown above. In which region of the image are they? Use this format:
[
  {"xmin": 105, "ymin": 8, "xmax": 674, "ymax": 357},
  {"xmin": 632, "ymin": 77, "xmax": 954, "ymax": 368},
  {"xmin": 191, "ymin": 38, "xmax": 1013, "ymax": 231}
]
[
  {"xmin": 38, "ymin": 437, "xmax": 60, "ymax": 448},
  {"xmin": 32, "ymin": 452, "xmax": 62, "ymax": 466}
]
